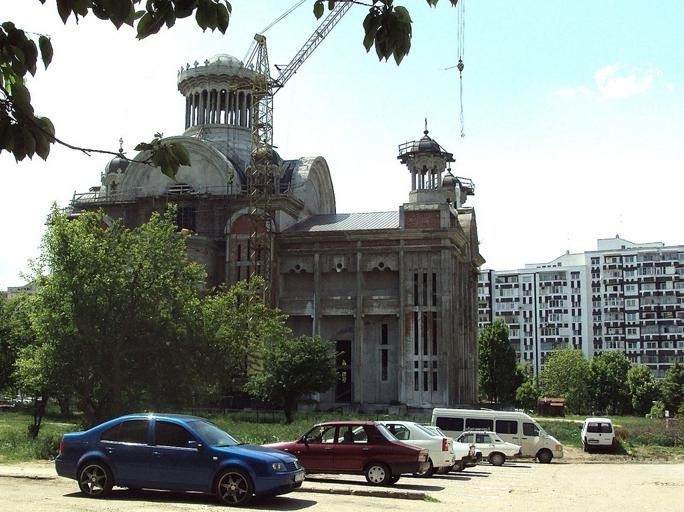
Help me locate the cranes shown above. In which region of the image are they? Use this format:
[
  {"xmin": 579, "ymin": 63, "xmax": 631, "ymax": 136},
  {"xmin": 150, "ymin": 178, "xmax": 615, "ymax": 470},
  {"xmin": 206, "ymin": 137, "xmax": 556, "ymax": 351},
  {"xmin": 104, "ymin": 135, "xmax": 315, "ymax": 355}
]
[{"xmin": 221, "ymin": 0, "xmax": 463, "ymax": 377}]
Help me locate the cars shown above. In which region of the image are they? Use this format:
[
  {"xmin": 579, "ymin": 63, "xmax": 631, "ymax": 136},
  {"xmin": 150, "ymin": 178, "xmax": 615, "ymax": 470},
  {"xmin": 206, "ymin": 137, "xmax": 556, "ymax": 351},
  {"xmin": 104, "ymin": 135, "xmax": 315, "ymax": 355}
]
[
  {"xmin": 0, "ymin": 393, "xmax": 43, "ymax": 412},
  {"xmin": 258, "ymin": 418, "xmax": 432, "ymax": 487},
  {"xmin": 51, "ymin": 411, "xmax": 310, "ymax": 509},
  {"xmin": 320, "ymin": 415, "xmax": 522, "ymax": 478}
]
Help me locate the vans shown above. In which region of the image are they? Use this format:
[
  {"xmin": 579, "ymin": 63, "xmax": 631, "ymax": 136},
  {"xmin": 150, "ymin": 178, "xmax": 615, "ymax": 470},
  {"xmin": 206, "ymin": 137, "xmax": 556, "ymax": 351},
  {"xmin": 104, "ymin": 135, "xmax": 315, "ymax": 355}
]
[
  {"xmin": 428, "ymin": 407, "xmax": 569, "ymax": 466},
  {"xmin": 578, "ymin": 416, "xmax": 617, "ymax": 453}
]
